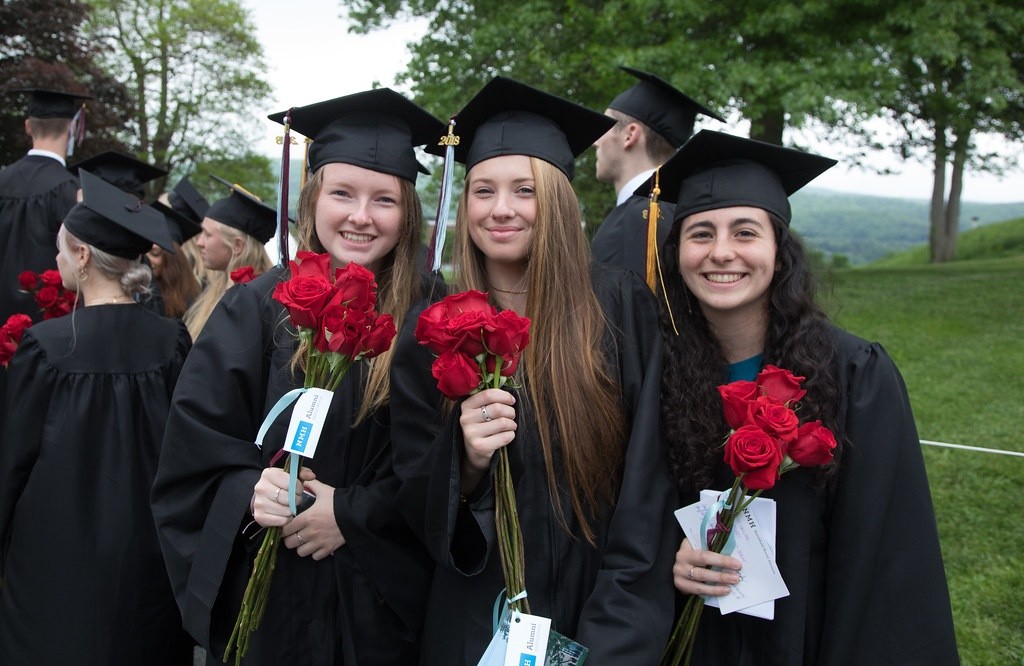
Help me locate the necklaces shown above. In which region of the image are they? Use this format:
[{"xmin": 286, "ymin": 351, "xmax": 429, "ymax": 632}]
[
  {"xmin": 106, "ymin": 294, "xmax": 128, "ymax": 304},
  {"xmin": 490, "ymin": 285, "xmax": 528, "ymax": 294}
]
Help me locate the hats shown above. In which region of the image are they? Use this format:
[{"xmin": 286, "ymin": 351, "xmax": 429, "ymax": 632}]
[
  {"xmin": 167, "ymin": 177, "xmax": 211, "ymax": 226},
  {"xmin": 63, "ymin": 149, "xmax": 167, "ymax": 188},
  {"xmin": 63, "ymin": 167, "xmax": 175, "ymax": 259},
  {"xmin": 267, "ymin": 88, "xmax": 444, "ymax": 268},
  {"xmin": 424, "ymin": 75, "xmax": 618, "ymax": 273},
  {"xmin": 203, "ymin": 175, "xmax": 296, "ymax": 245},
  {"xmin": 10, "ymin": 89, "xmax": 97, "ymax": 155},
  {"xmin": 611, "ymin": 65, "xmax": 726, "ymax": 148},
  {"xmin": 633, "ymin": 130, "xmax": 839, "ymax": 334}
]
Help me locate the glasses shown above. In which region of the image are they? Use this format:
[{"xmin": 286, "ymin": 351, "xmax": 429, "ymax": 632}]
[{"xmin": 150, "ymin": 245, "xmax": 163, "ymax": 256}]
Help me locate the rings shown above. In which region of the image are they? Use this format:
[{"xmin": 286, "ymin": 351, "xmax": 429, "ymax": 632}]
[
  {"xmin": 688, "ymin": 566, "xmax": 695, "ymax": 581},
  {"xmin": 296, "ymin": 532, "xmax": 305, "ymax": 545},
  {"xmin": 275, "ymin": 488, "xmax": 282, "ymax": 503},
  {"xmin": 481, "ymin": 405, "xmax": 491, "ymax": 421}
]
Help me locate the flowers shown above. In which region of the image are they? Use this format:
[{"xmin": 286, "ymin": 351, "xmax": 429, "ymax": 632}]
[
  {"xmin": 659, "ymin": 363, "xmax": 837, "ymax": 666},
  {"xmin": 0, "ymin": 269, "xmax": 80, "ymax": 369},
  {"xmin": 413, "ymin": 289, "xmax": 533, "ymax": 615},
  {"xmin": 228, "ymin": 266, "xmax": 257, "ymax": 286},
  {"xmin": 222, "ymin": 250, "xmax": 399, "ymax": 666}
]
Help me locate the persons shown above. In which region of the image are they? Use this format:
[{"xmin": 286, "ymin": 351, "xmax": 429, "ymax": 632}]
[
  {"xmin": 588, "ymin": 64, "xmax": 726, "ymax": 287},
  {"xmin": 150, "ymin": 87, "xmax": 448, "ymax": 666},
  {"xmin": 182, "ymin": 174, "xmax": 295, "ymax": 343},
  {"xmin": 72, "ymin": 149, "xmax": 210, "ymax": 317},
  {"xmin": 633, "ymin": 130, "xmax": 961, "ymax": 666},
  {"xmin": 0, "ymin": 88, "xmax": 93, "ymax": 322},
  {"xmin": 423, "ymin": 76, "xmax": 681, "ymax": 666},
  {"xmin": 0, "ymin": 166, "xmax": 194, "ymax": 666}
]
